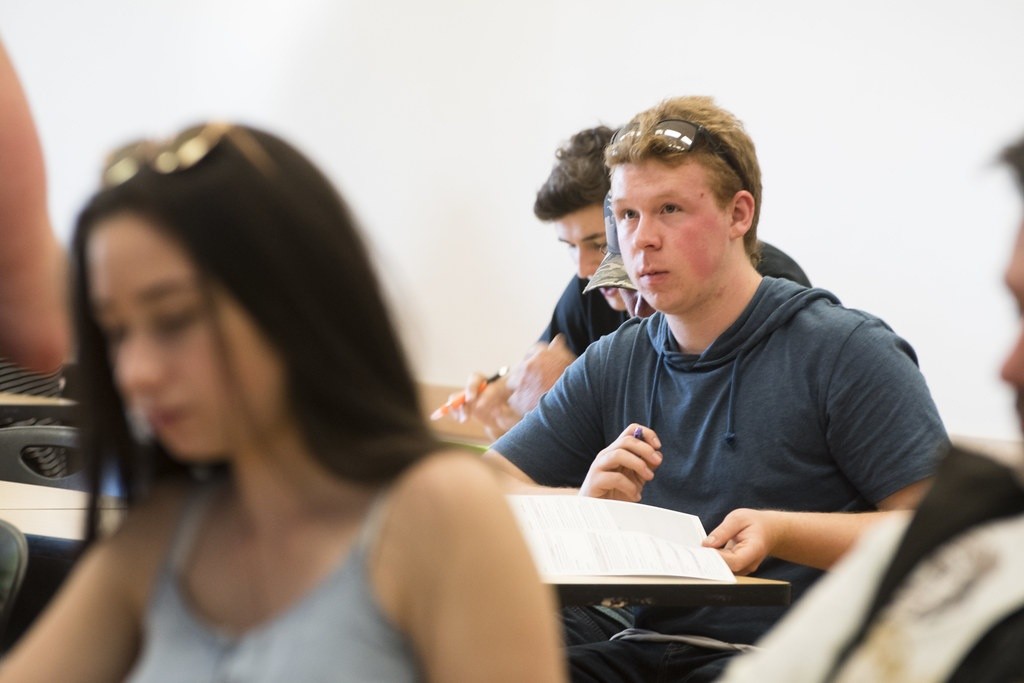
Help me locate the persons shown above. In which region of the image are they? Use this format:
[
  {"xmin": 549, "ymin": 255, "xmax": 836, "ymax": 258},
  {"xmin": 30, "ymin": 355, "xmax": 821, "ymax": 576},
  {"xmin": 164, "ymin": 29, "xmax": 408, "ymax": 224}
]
[
  {"xmin": 446, "ymin": 123, "xmax": 813, "ymax": 442},
  {"xmin": 479, "ymin": 99, "xmax": 954, "ymax": 683},
  {"xmin": 716, "ymin": 136, "xmax": 1024, "ymax": 683},
  {"xmin": 0, "ymin": 125, "xmax": 567, "ymax": 683},
  {"xmin": 1, "ymin": 37, "xmax": 74, "ymax": 477}
]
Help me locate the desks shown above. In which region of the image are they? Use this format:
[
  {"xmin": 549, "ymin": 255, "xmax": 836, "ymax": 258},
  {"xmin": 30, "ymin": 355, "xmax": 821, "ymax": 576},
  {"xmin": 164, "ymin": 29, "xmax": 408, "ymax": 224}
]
[
  {"xmin": 553, "ymin": 574, "xmax": 789, "ymax": 603},
  {"xmin": 0, "ymin": 391, "xmax": 77, "ymax": 423}
]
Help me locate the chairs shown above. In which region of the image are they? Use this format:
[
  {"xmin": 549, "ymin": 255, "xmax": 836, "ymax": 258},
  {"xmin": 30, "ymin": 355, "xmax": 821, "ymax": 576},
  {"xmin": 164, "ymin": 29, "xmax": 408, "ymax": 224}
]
[
  {"xmin": 423, "ymin": 382, "xmax": 486, "ymax": 439},
  {"xmin": 0, "ymin": 426, "xmax": 87, "ymax": 545}
]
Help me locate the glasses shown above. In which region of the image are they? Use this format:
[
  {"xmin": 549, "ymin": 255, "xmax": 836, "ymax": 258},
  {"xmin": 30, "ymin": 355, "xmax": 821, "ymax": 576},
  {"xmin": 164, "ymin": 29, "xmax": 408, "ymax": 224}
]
[
  {"xmin": 613, "ymin": 117, "xmax": 754, "ymax": 196},
  {"xmin": 102, "ymin": 121, "xmax": 271, "ymax": 191}
]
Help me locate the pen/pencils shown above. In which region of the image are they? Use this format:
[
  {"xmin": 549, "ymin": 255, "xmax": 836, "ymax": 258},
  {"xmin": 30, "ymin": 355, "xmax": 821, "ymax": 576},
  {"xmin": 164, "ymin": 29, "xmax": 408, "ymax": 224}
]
[
  {"xmin": 430, "ymin": 365, "xmax": 512, "ymax": 421},
  {"xmin": 634, "ymin": 427, "xmax": 644, "ymax": 440}
]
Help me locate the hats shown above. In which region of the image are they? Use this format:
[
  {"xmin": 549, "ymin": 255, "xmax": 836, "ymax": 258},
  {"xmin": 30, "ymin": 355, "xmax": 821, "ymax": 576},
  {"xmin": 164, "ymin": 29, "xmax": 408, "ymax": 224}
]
[{"xmin": 581, "ymin": 189, "xmax": 638, "ymax": 294}]
